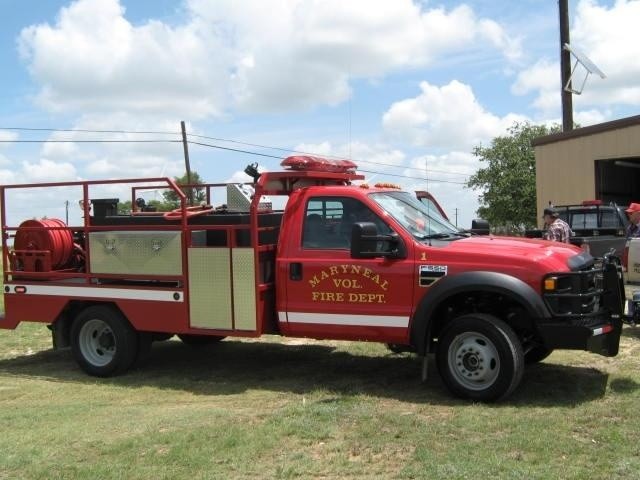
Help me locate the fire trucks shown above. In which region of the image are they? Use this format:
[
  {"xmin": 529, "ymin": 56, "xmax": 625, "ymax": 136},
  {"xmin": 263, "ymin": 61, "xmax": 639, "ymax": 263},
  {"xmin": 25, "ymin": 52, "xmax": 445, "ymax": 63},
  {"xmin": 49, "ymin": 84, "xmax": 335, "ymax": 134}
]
[{"xmin": 1, "ymin": 154, "xmax": 626, "ymax": 403}]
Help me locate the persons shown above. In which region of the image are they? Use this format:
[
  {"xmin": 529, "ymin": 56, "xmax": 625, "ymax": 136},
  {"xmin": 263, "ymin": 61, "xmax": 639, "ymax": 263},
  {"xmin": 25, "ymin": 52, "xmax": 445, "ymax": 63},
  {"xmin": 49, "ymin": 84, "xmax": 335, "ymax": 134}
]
[
  {"xmin": 621, "ymin": 201, "xmax": 640, "ymax": 326},
  {"xmin": 542, "ymin": 208, "xmax": 573, "ymax": 245}
]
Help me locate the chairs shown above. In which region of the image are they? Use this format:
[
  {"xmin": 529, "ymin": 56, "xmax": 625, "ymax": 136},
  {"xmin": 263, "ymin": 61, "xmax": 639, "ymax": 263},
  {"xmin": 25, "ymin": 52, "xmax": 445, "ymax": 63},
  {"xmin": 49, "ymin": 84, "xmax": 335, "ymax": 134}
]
[{"xmin": 303, "ymin": 214, "xmax": 349, "ymax": 249}]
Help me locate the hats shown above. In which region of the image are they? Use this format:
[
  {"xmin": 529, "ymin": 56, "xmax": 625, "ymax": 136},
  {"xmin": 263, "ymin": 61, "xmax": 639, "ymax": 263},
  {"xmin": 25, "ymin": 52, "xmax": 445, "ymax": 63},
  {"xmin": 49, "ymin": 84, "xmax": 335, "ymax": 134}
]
[
  {"xmin": 624, "ymin": 202, "xmax": 640, "ymax": 213},
  {"xmin": 540, "ymin": 207, "xmax": 559, "ymax": 218}
]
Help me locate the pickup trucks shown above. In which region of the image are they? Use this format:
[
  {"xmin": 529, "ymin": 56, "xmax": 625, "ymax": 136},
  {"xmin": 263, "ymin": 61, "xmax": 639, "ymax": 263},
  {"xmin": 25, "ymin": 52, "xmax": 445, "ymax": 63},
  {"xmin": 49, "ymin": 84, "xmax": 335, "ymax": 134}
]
[{"xmin": 524, "ymin": 199, "xmax": 630, "ymax": 266}]
